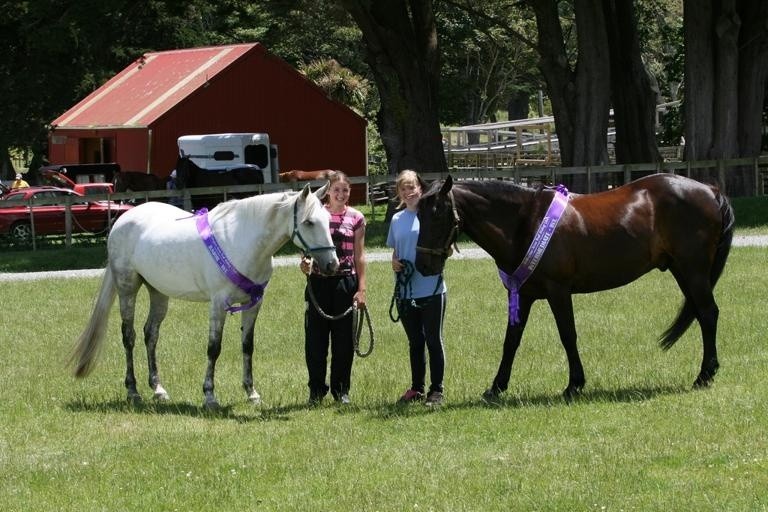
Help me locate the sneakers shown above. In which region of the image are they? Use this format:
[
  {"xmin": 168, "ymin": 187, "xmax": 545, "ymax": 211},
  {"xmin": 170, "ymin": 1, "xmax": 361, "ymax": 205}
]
[
  {"xmin": 335, "ymin": 393, "xmax": 349, "ymax": 404},
  {"xmin": 424, "ymin": 390, "xmax": 442, "ymax": 406},
  {"xmin": 309, "ymin": 386, "xmax": 328, "ymax": 404},
  {"xmin": 397, "ymin": 389, "xmax": 426, "ymax": 404}
]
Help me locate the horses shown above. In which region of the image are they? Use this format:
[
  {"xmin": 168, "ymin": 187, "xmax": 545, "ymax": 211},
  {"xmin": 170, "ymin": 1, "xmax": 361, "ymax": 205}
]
[
  {"xmin": 411, "ymin": 170, "xmax": 737, "ymax": 408},
  {"xmin": 61, "ymin": 181, "xmax": 344, "ymax": 411},
  {"xmin": 278, "ymin": 169, "xmax": 339, "ymax": 182},
  {"xmin": 174, "ymin": 154, "xmax": 265, "ymax": 211},
  {"xmin": 110, "ymin": 168, "xmax": 170, "ymax": 204}
]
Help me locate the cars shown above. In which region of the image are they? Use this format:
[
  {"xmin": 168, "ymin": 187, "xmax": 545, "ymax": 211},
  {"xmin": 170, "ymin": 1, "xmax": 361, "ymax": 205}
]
[{"xmin": 0, "ymin": 159, "xmax": 140, "ymax": 243}]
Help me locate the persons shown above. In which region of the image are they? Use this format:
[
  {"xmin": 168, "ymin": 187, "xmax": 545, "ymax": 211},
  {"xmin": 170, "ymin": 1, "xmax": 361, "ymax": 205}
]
[
  {"xmin": 165, "ymin": 168, "xmax": 183, "ymax": 209},
  {"xmin": 10, "ymin": 173, "xmax": 29, "ymax": 188},
  {"xmin": 295, "ymin": 169, "xmax": 367, "ymax": 408},
  {"xmin": 381, "ymin": 167, "xmax": 450, "ymax": 408}
]
